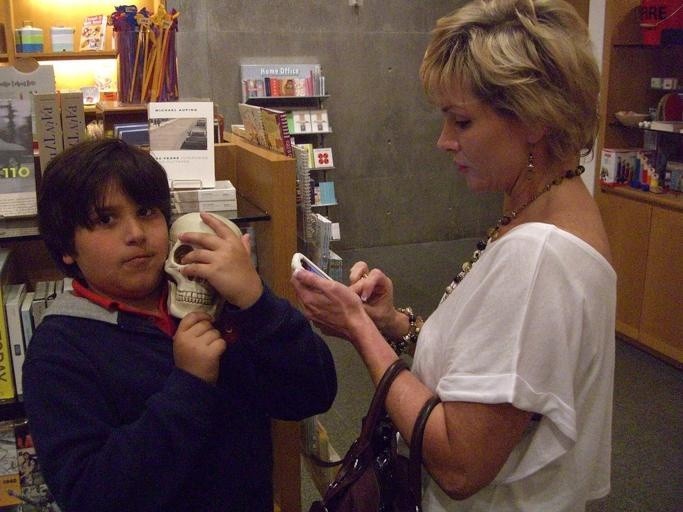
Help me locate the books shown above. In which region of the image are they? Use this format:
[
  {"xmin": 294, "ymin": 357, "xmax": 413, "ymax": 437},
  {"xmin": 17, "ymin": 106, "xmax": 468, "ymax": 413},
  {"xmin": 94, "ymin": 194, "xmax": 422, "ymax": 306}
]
[
  {"xmin": 1, "ymin": 82, "xmax": 233, "ymax": 222},
  {"xmin": 79, "ymin": 14, "xmax": 108, "ymax": 51},
  {"xmin": 240, "ymin": 61, "xmax": 345, "ymax": 285},
  {"xmin": 0, "ymin": 266, "xmax": 69, "ymax": 511}
]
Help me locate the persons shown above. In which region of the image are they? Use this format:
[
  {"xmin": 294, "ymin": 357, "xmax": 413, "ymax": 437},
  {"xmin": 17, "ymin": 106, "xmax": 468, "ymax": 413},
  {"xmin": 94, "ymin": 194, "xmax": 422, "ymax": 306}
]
[
  {"xmin": 15, "ymin": 144, "xmax": 338, "ymax": 512},
  {"xmin": 287, "ymin": 0, "xmax": 619, "ymax": 512}
]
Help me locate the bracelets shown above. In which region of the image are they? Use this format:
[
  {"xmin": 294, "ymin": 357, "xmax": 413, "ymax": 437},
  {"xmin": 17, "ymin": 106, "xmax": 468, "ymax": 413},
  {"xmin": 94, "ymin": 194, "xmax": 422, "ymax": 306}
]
[{"xmin": 384, "ymin": 303, "xmax": 420, "ymax": 349}]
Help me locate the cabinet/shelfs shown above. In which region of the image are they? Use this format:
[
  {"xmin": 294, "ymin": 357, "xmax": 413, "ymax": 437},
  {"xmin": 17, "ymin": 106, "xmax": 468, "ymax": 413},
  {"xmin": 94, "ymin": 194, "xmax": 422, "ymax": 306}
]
[
  {"xmin": 0, "ymin": 0, "xmax": 333, "ymax": 512},
  {"xmin": 592, "ymin": 0, "xmax": 683, "ymax": 367}
]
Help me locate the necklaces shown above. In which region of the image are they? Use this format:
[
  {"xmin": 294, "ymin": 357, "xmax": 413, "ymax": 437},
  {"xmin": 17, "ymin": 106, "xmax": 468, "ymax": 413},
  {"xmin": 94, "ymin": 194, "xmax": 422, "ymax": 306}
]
[{"xmin": 439, "ymin": 148, "xmax": 583, "ymax": 300}]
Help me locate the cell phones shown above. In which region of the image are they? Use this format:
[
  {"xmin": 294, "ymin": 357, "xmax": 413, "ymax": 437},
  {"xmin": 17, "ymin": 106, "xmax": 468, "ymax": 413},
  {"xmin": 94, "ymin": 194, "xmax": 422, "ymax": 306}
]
[{"xmin": 290, "ymin": 252, "xmax": 333, "ymax": 282}]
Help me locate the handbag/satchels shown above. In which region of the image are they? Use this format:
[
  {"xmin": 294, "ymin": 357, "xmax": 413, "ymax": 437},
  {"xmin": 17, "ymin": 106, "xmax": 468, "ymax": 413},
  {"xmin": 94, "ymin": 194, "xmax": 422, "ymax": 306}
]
[{"xmin": 311, "ymin": 415, "xmax": 423, "ymax": 512}]
